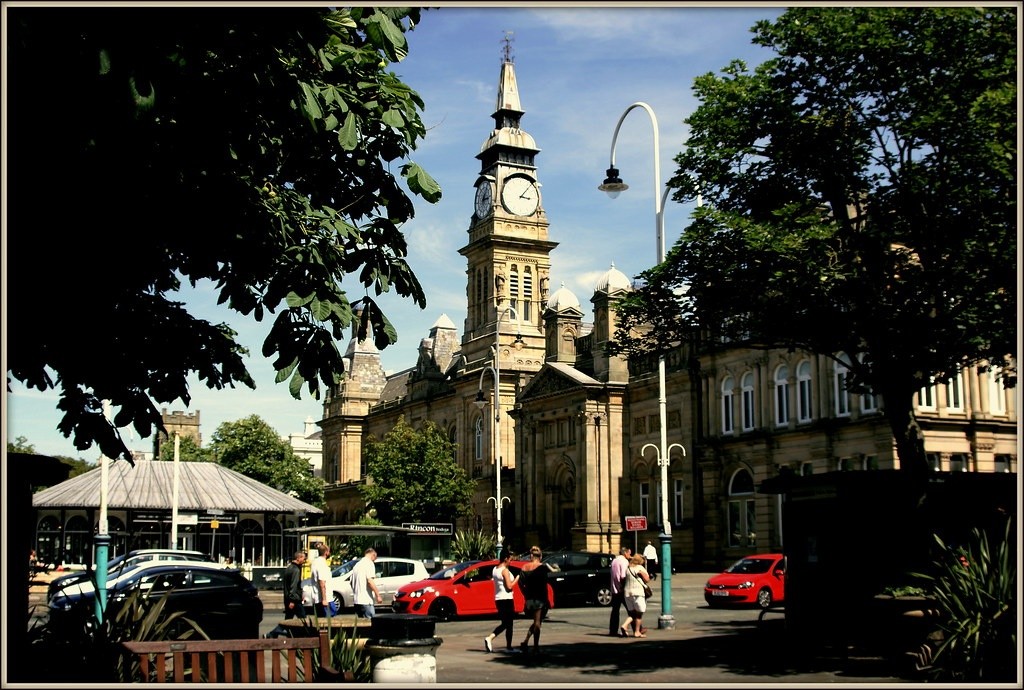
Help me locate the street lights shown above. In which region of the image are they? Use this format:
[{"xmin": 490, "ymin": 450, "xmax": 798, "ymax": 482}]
[
  {"xmin": 599, "ymin": 98, "xmax": 707, "ymax": 627},
  {"xmin": 474, "ymin": 306, "xmax": 528, "ymax": 557}
]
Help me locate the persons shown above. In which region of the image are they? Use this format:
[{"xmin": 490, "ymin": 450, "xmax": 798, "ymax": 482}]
[
  {"xmin": 496, "ymin": 265, "xmax": 508, "ymax": 296},
  {"xmin": 212, "ymin": 555, "xmax": 253, "ymax": 582},
  {"xmin": 642, "ymin": 540, "xmax": 658, "ymax": 580},
  {"xmin": 341, "ymin": 553, "xmax": 357, "ymax": 564},
  {"xmin": 499, "ymin": 543, "xmax": 513, "ymax": 563},
  {"xmin": 541, "ymin": 270, "xmax": 550, "ymax": 298},
  {"xmin": 29, "ymin": 550, "xmax": 38, "ymax": 576},
  {"xmin": 485, "ymin": 551, "xmax": 522, "ymax": 653},
  {"xmin": 263, "ymin": 551, "xmax": 307, "ymax": 640},
  {"xmin": 375, "ymin": 565, "xmax": 383, "ymax": 578},
  {"xmin": 609, "ymin": 546, "xmax": 647, "ymax": 637},
  {"xmin": 152, "ymin": 572, "xmax": 187, "ymax": 591},
  {"xmin": 350, "ymin": 547, "xmax": 383, "ymax": 618},
  {"xmin": 519, "ymin": 546, "xmax": 561, "ymax": 650},
  {"xmin": 311, "ymin": 545, "xmax": 336, "ymax": 621},
  {"xmin": 621, "ymin": 553, "xmax": 650, "ymax": 637},
  {"xmin": 485, "ymin": 571, "xmax": 494, "ymax": 580}
]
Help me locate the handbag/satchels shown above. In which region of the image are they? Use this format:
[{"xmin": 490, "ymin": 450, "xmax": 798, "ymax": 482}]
[{"xmin": 644, "ymin": 585, "xmax": 652, "ymax": 599}]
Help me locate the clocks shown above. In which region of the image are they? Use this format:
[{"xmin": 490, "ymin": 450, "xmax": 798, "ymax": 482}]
[
  {"xmin": 501, "ymin": 176, "xmax": 539, "ymax": 217},
  {"xmin": 473, "ymin": 179, "xmax": 496, "ymax": 220}
]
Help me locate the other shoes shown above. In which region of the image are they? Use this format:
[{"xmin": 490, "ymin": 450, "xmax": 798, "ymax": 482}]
[
  {"xmin": 609, "ymin": 631, "xmax": 621, "ymax": 637},
  {"xmin": 520, "ymin": 642, "xmax": 528, "ymax": 650},
  {"xmin": 640, "ymin": 628, "xmax": 646, "ymax": 634},
  {"xmin": 485, "ymin": 637, "xmax": 493, "ymax": 653},
  {"xmin": 505, "ymin": 648, "xmax": 520, "ymax": 652},
  {"xmin": 621, "ymin": 626, "xmax": 629, "ymax": 637},
  {"xmin": 634, "ymin": 633, "xmax": 646, "ymax": 637},
  {"xmin": 532, "ymin": 648, "xmax": 540, "ymax": 652},
  {"xmin": 654, "ymin": 575, "xmax": 657, "ymax": 581}
]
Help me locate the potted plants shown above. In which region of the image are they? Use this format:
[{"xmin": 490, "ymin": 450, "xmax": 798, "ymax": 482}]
[{"xmin": 874, "ymin": 583, "xmax": 950, "ymax": 677}]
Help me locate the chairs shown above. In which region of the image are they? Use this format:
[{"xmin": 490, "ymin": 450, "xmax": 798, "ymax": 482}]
[{"xmin": 388, "ymin": 565, "xmax": 402, "ymax": 576}]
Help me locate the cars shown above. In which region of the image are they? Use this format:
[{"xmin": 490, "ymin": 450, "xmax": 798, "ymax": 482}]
[
  {"xmin": 391, "ymin": 558, "xmax": 555, "ymax": 618},
  {"xmin": 329, "ymin": 557, "xmax": 430, "ymax": 612},
  {"xmin": 702, "ymin": 553, "xmax": 785, "ymax": 608},
  {"xmin": 522, "ymin": 550, "xmax": 615, "ymax": 607}
]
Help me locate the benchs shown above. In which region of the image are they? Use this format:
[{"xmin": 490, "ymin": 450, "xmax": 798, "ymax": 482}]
[{"xmin": 278, "ymin": 617, "xmax": 372, "ymax": 638}]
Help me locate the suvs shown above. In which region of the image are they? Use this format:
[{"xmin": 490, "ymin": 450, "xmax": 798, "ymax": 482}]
[{"xmin": 47, "ymin": 547, "xmax": 263, "ymax": 643}]
